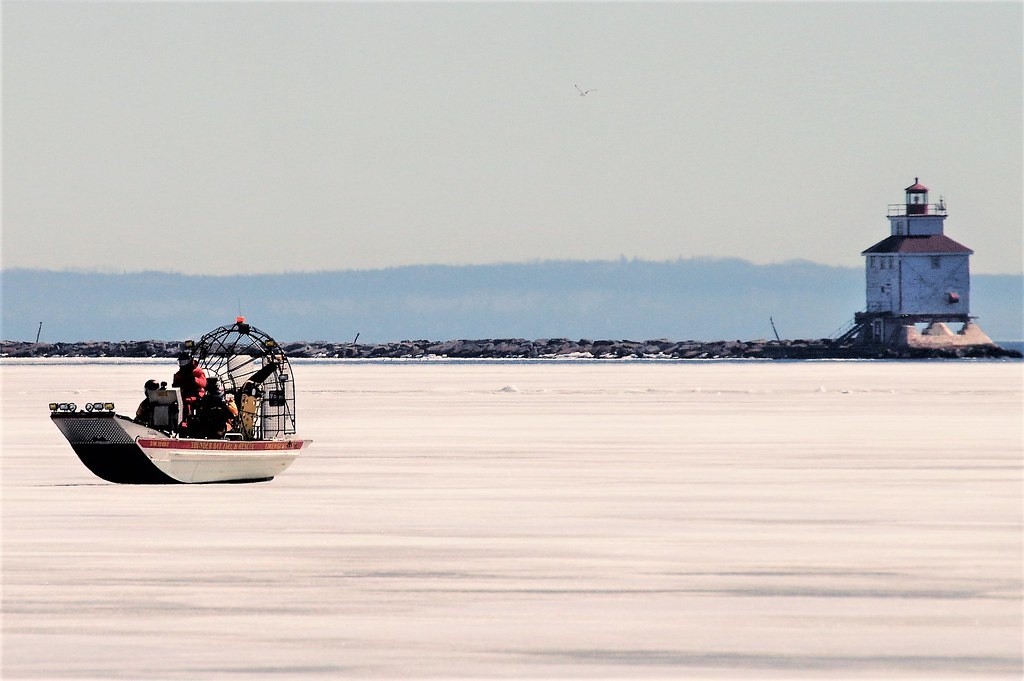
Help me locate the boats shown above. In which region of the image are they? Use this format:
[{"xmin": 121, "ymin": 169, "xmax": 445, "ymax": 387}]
[{"xmin": 48, "ymin": 319, "xmax": 314, "ymax": 486}]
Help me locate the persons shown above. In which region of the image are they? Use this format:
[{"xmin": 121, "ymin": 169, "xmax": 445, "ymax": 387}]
[
  {"xmin": 136, "ymin": 379, "xmax": 160, "ymax": 425},
  {"xmin": 179, "ymin": 378, "xmax": 238, "ymax": 439},
  {"xmin": 172, "ymin": 352, "xmax": 206, "ymax": 427}
]
